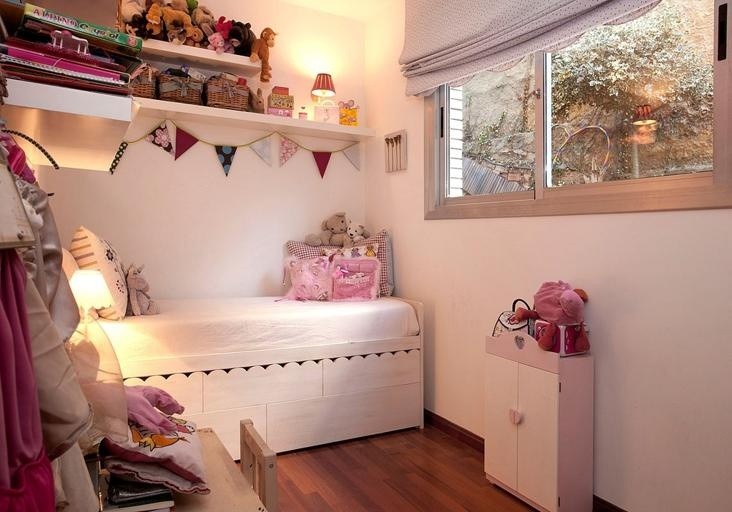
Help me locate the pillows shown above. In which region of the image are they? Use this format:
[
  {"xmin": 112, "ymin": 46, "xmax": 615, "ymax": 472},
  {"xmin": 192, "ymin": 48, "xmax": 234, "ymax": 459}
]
[
  {"xmin": 284, "ymin": 228, "xmax": 397, "ymax": 300},
  {"xmin": 67, "ymin": 227, "xmax": 130, "ymax": 321}
]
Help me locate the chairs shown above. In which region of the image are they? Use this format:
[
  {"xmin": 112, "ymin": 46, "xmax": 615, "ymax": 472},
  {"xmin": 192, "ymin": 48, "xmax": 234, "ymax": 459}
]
[{"xmin": 232, "ymin": 419, "xmax": 277, "ymax": 511}]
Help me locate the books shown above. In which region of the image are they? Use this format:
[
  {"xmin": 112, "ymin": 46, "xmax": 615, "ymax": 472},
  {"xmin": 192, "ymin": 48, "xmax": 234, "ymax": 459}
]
[{"xmin": 106, "ymin": 475, "xmax": 174, "ymax": 512}]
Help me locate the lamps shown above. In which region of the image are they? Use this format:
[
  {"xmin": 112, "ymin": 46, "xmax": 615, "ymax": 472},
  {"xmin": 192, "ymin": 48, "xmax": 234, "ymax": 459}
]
[
  {"xmin": 311, "ymin": 73, "xmax": 335, "ymax": 120},
  {"xmin": 66, "ymin": 269, "xmax": 117, "ymax": 366}
]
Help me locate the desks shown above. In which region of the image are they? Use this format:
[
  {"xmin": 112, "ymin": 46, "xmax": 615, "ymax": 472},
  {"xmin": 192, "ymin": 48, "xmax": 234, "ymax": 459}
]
[{"xmin": 99, "ymin": 428, "xmax": 268, "ymax": 512}]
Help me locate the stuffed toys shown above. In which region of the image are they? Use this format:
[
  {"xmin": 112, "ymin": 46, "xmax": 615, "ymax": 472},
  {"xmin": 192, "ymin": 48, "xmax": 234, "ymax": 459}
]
[
  {"xmin": 248, "ymin": 87, "xmax": 264, "ymax": 113},
  {"xmin": 344, "ymin": 219, "xmax": 365, "ymax": 242},
  {"xmin": 119, "ymin": 0, "xmax": 277, "ymax": 82},
  {"xmin": 126, "ymin": 263, "xmax": 158, "ymax": 315},
  {"xmin": 305, "ymin": 212, "xmax": 371, "ymax": 248}
]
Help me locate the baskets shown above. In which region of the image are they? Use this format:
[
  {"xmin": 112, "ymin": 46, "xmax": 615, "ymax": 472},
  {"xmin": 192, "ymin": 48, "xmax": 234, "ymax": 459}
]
[
  {"xmin": 202, "ymin": 76, "xmax": 249, "ymax": 111},
  {"xmin": 133, "ymin": 66, "xmax": 159, "ymax": 97},
  {"xmin": 159, "ymin": 71, "xmax": 201, "ymax": 107}
]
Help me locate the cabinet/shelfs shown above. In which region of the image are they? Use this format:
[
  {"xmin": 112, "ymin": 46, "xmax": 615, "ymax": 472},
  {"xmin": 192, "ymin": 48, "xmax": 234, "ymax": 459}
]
[
  {"xmin": 0, "ymin": 0, "xmax": 374, "ymax": 143},
  {"xmin": 484, "ymin": 331, "xmax": 593, "ymax": 512}
]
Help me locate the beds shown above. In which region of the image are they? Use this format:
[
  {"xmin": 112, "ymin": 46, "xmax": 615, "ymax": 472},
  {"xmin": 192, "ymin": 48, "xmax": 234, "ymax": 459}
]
[{"xmin": 60, "ymin": 293, "xmax": 424, "ymax": 463}]
